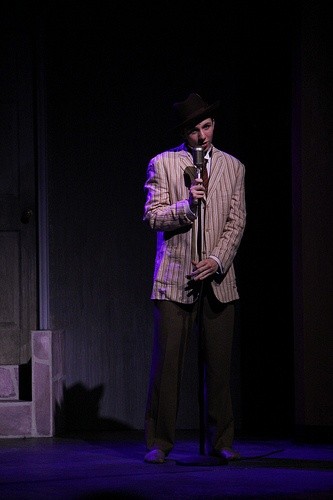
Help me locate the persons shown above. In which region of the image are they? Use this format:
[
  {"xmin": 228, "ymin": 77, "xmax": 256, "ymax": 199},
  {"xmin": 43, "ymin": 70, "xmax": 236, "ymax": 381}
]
[{"xmin": 145, "ymin": 93, "xmax": 246, "ymax": 466}]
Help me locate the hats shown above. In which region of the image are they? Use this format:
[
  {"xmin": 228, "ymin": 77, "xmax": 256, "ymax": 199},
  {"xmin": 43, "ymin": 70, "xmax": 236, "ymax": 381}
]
[{"xmin": 168, "ymin": 92, "xmax": 220, "ymax": 131}]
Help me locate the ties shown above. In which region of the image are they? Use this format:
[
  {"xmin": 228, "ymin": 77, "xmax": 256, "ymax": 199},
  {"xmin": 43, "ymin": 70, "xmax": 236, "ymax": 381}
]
[{"xmin": 199, "ymin": 158, "xmax": 211, "ymax": 198}]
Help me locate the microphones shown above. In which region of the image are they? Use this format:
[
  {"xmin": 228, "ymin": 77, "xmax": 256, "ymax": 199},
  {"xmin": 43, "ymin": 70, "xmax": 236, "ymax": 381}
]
[{"xmin": 193, "ymin": 147, "xmax": 203, "ymax": 166}]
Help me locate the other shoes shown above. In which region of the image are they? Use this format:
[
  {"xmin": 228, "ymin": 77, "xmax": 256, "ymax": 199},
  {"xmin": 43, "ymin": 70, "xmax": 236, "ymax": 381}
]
[
  {"xmin": 145, "ymin": 450, "xmax": 166, "ymax": 463},
  {"xmin": 220, "ymin": 447, "xmax": 239, "ymax": 460}
]
